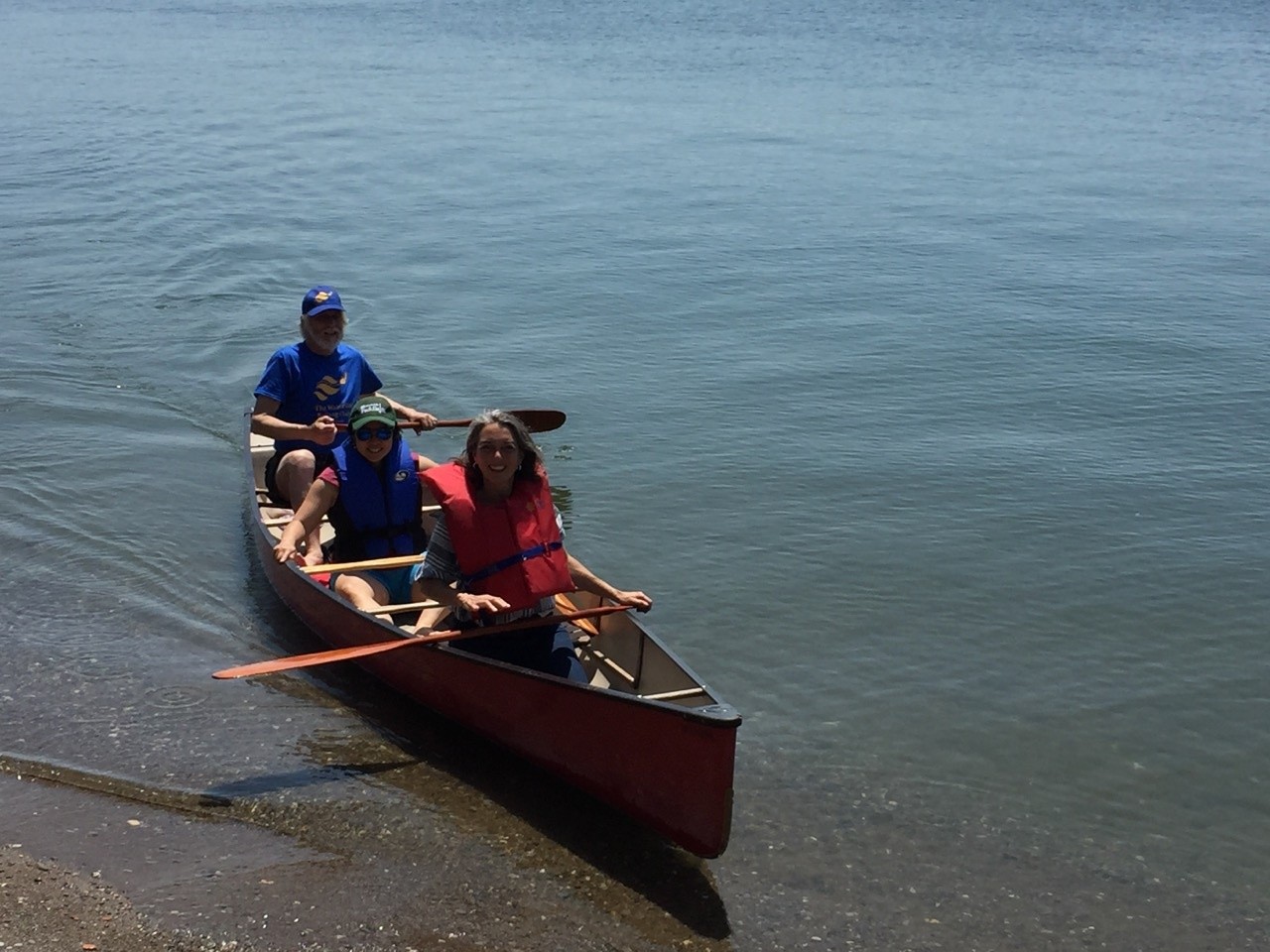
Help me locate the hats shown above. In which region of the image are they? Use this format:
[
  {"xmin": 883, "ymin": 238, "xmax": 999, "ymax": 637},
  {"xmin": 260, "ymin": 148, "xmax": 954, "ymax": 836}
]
[
  {"xmin": 302, "ymin": 285, "xmax": 346, "ymax": 317},
  {"xmin": 347, "ymin": 396, "xmax": 395, "ymax": 430}
]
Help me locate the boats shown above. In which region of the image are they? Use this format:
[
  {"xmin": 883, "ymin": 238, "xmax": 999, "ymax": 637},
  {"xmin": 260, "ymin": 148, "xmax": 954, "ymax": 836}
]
[{"xmin": 242, "ymin": 405, "xmax": 744, "ymax": 860}]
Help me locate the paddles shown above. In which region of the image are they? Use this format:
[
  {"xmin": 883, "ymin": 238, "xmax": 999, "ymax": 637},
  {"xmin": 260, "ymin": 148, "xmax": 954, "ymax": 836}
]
[
  {"xmin": 330, "ymin": 408, "xmax": 570, "ymax": 434},
  {"xmin": 212, "ymin": 599, "xmax": 635, "ymax": 682}
]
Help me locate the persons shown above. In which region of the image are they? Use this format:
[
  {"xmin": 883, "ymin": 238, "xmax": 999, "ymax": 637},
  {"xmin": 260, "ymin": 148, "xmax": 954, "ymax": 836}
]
[
  {"xmin": 413, "ymin": 411, "xmax": 653, "ymax": 683},
  {"xmin": 271, "ymin": 396, "xmax": 452, "ymax": 638},
  {"xmin": 250, "ymin": 286, "xmax": 437, "ymax": 566}
]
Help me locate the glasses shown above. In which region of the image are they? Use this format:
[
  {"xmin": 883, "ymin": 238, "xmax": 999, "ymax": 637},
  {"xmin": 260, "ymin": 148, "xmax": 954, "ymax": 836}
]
[
  {"xmin": 474, "ymin": 443, "xmax": 520, "ymax": 455},
  {"xmin": 355, "ymin": 428, "xmax": 391, "ymax": 441}
]
